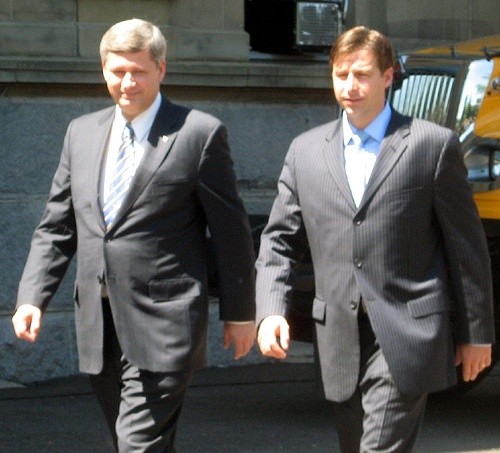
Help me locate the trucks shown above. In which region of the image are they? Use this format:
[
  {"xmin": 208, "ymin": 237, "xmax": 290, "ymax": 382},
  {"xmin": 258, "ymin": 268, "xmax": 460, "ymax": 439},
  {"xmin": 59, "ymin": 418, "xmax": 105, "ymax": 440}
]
[{"xmin": 391, "ymin": 32, "xmax": 500, "ymax": 262}]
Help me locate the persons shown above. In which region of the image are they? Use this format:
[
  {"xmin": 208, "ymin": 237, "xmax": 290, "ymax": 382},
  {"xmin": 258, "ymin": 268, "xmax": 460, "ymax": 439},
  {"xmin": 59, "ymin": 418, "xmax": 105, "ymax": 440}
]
[
  {"xmin": 11, "ymin": 18, "xmax": 256, "ymax": 453},
  {"xmin": 255, "ymin": 24, "xmax": 495, "ymax": 453}
]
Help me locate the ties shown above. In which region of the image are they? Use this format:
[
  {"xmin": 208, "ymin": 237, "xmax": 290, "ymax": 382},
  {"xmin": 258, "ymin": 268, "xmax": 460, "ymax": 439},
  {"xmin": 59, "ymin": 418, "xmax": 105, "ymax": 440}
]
[
  {"xmin": 348, "ymin": 129, "xmax": 371, "ymax": 205},
  {"xmin": 101, "ymin": 125, "xmax": 135, "ymax": 229}
]
